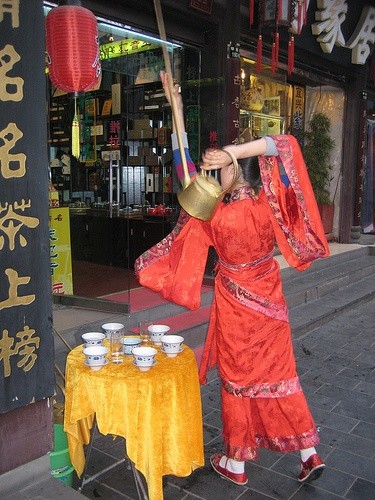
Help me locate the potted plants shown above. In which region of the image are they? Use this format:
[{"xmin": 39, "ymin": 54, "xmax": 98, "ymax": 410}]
[{"xmin": 298, "ymin": 112, "xmax": 335, "ymax": 234}]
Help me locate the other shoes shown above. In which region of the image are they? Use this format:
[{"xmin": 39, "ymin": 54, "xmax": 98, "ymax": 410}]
[
  {"xmin": 298, "ymin": 454, "xmax": 325, "ymax": 482},
  {"xmin": 210, "ymin": 452, "xmax": 249, "ymax": 485}
]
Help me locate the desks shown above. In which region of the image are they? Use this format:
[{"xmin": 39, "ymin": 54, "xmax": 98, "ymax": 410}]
[{"xmin": 65, "ymin": 336, "xmax": 205, "ymax": 500}]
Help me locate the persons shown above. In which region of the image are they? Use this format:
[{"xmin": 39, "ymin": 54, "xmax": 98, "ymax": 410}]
[{"xmin": 134, "ymin": 70, "xmax": 331, "ymax": 486}]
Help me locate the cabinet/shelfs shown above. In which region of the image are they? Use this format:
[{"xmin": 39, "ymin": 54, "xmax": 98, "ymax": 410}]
[{"xmin": 48, "ymin": 47, "xmax": 173, "ymax": 270}]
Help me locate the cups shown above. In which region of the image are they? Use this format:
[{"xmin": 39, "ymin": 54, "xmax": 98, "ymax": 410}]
[
  {"xmin": 147, "ymin": 323, "xmax": 170, "ymax": 339},
  {"xmin": 131, "ymin": 347, "xmax": 158, "ymax": 364},
  {"xmin": 101, "ymin": 323, "xmax": 124, "ymax": 338},
  {"xmin": 161, "ymin": 335, "xmax": 185, "ymax": 352},
  {"xmin": 110, "ymin": 331, "xmax": 124, "ymax": 364},
  {"xmin": 139, "ymin": 319, "xmax": 155, "ymax": 346},
  {"xmin": 81, "ymin": 332, "xmax": 105, "ymax": 346},
  {"xmin": 121, "ymin": 337, "xmax": 142, "ymax": 355},
  {"xmin": 82, "ymin": 346, "xmax": 109, "ymax": 364}
]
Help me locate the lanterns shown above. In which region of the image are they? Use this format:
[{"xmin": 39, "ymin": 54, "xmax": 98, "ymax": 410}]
[
  {"xmin": 249, "ymin": 0, "xmax": 309, "ymax": 76},
  {"xmin": 46, "ymin": 0, "xmax": 100, "ymax": 157}
]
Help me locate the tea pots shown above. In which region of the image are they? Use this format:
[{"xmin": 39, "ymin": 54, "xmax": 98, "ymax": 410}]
[{"xmin": 154, "ymin": 0, "xmax": 238, "ymax": 221}]
